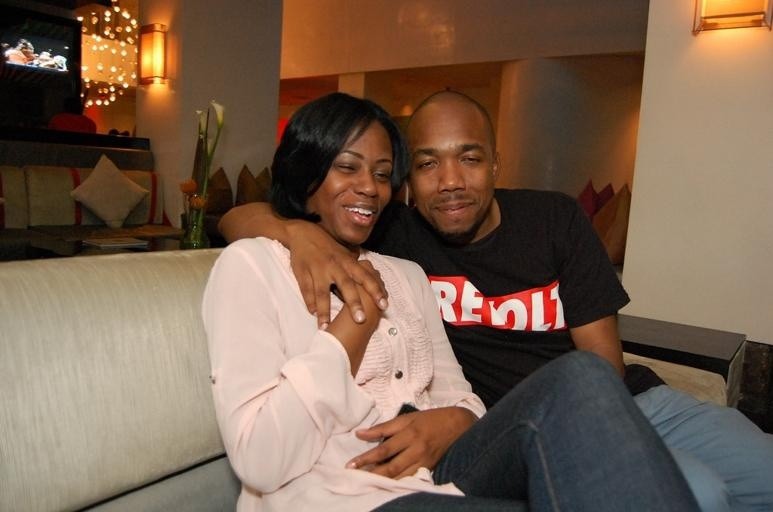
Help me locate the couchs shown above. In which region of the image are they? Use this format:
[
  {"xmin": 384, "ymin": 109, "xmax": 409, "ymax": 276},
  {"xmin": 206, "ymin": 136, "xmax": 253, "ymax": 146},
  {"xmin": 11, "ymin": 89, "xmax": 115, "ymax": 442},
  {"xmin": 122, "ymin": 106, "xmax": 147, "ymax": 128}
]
[
  {"xmin": 0, "ymin": 245, "xmax": 748, "ymax": 512},
  {"xmin": 1, "ymin": 143, "xmax": 187, "ymax": 258}
]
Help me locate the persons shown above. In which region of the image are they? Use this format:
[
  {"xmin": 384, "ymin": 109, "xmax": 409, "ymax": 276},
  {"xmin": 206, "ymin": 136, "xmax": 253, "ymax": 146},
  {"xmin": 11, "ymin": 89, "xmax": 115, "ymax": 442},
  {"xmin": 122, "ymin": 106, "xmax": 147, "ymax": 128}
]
[{"xmin": 0, "ymin": 39, "xmax": 67, "ymax": 71}]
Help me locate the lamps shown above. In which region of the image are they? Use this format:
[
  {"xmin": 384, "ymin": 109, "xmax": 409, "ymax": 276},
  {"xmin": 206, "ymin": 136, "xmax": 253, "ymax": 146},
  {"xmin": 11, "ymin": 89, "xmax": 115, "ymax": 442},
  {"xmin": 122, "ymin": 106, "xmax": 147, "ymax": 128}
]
[
  {"xmin": 135, "ymin": 24, "xmax": 168, "ymax": 86},
  {"xmin": 695, "ymin": 3, "xmax": 772, "ymax": 32}
]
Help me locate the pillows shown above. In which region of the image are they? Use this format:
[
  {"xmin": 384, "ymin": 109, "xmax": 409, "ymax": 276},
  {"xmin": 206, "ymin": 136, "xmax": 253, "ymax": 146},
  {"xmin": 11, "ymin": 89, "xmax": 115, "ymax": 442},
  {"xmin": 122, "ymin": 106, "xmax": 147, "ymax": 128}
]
[{"xmin": 70, "ymin": 155, "xmax": 149, "ymax": 231}]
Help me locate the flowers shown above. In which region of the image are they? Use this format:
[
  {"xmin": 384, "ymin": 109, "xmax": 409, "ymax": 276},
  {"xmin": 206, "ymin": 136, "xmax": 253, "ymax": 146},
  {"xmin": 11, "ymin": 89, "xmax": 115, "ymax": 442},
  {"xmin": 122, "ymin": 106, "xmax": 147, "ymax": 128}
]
[{"xmin": 179, "ymin": 99, "xmax": 226, "ymax": 243}]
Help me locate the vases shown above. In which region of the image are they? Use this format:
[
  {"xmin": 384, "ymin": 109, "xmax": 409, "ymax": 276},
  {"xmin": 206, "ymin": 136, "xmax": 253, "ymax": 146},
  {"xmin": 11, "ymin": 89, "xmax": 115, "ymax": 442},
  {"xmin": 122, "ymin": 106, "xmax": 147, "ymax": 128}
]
[{"xmin": 185, "ymin": 202, "xmax": 211, "ymax": 251}]
